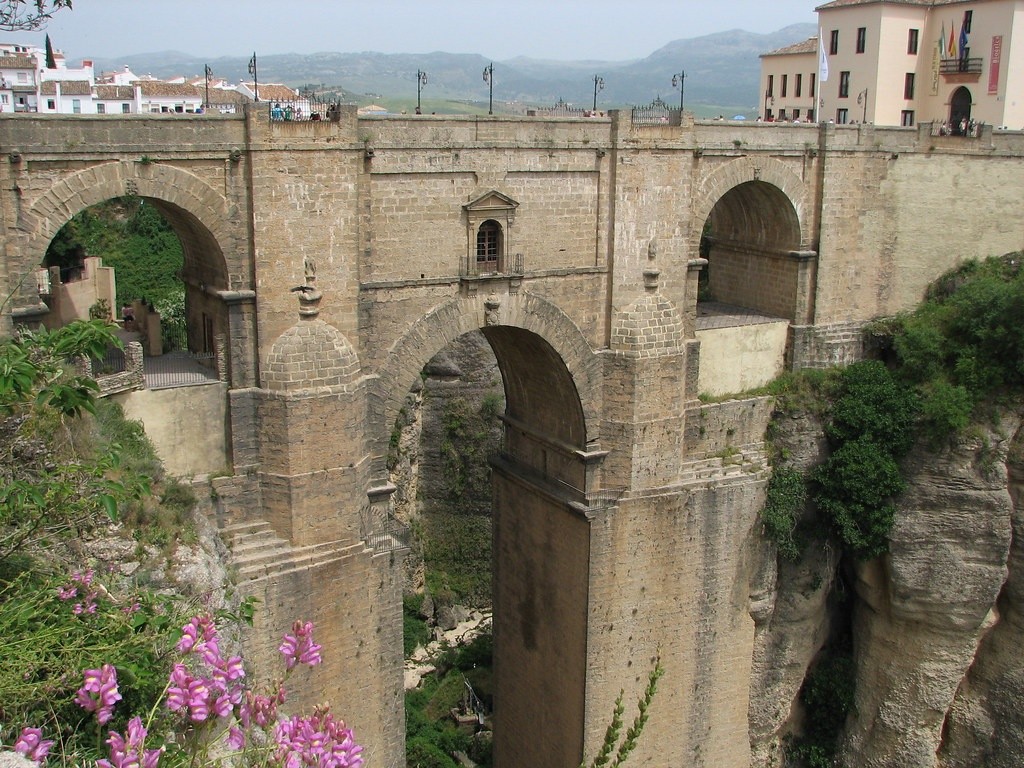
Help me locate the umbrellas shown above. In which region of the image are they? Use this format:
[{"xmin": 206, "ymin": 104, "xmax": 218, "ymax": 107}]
[{"xmin": 732, "ymin": 115, "xmax": 745, "ymax": 120}]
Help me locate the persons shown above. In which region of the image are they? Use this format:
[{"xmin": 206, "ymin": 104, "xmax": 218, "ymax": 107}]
[
  {"xmin": 271, "ymin": 104, "xmax": 303, "ymax": 122},
  {"xmin": 941, "ymin": 118, "xmax": 967, "ymax": 137},
  {"xmin": 794, "ymin": 118, "xmax": 800, "ymax": 123},
  {"xmin": 806, "ymin": 116, "xmax": 812, "ymax": 123},
  {"xmin": 327, "ymin": 104, "xmax": 337, "ymax": 121},
  {"xmin": 195, "ymin": 104, "xmax": 205, "ymax": 113},
  {"xmin": 849, "ymin": 120, "xmax": 873, "ymax": 125},
  {"xmin": 783, "ymin": 117, "xmax": 788, "ymax": 123},
  {"xmin": 309, "ymin": 110, "xmax": 321, "ymax": 122},
  {"xmin": 768, "ymin": 115, "xmax": 775, "ymax": 122},
  {"xmin": 829, "ymin": 118, "xmax": 834, "ymax": 124},
  {"xmin": 121, "ymin": 303, "xmax": 135, "ymax": 332},
  {"xmin": 719, "ymin": 114, "xmax": 723, "ymax": 120},
  {"xmin": 589, "ymin": 107, "xmax": 604, "ymax": 118},
  {"xmin": 756, "ymin": 116, "xmax": 761, "ymax": 122}
]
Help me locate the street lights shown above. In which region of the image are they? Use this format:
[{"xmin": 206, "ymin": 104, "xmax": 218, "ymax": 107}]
[
  {"xmin": 858, "ymin": 88, "xmax": 868, "ymax": 124},
  {"xmin": 248, "ymin": 52, "xmax": 259, "ymax": 102},
  {"xmin": 483, "ymin": 62, "xmax": 495, "ymax": 115},
  {"xmin": 764, "ymin": 90, "xmax": 775, "ymax": 121},
  {"xmin": 205, "ymin": 64, "xmax": 213, "ymax": 108},
  {"xmin": 593, "ymin": 74, "xmax": 604, "ymax": 112},
  {"xmin": 672, "ymin": 70, "xmax": 684, "ymax": 110},
  {"xmin": 415, "ymin": 69, "xmax": 427, "ymax": 114},
  {"xmin": 813, "ymin": 96, "xmax": 824, "ymax": 123}
]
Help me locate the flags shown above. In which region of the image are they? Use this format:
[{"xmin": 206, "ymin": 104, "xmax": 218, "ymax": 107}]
[
  {"xmin": 939, "ymin": 29, "xmax": 947, "ymax": 59},
  {"xmin": 948, "ymin": 31, "xmax": 957, "ymax": 57},
  {"xmin": 820, "ymin": 28, "xmax": 828, "ymax": 82},
  {"xmin": 958, "ymin": 26, "xmax": 968, "ymax": 59}
]
